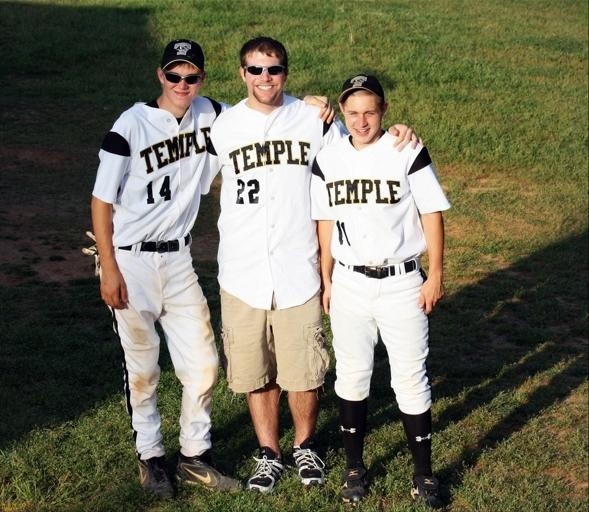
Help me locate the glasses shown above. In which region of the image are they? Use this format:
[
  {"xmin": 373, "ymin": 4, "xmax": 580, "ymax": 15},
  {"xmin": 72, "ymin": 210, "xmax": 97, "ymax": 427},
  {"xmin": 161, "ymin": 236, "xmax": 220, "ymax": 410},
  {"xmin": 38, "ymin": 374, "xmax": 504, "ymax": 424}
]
[
  {"xmin": 244, "ymin": 64, "xmax": 286, "ymax": 76},
  {"xmin": 162, "ymin": 72, "xmax": 202, "ymax": 85}
]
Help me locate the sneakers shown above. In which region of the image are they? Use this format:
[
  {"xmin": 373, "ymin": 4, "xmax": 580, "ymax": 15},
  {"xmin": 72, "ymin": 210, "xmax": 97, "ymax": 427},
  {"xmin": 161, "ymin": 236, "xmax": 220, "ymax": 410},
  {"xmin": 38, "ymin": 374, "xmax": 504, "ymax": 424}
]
[
  {"xmin": 291, "ymin": 445, "xmax": 326, "ymax": 486},
  {"xmin": 246, "ymin": 446, "xmax": 285, "ymax": 493},
  {"xmin": 176, "ymin": 449, "xmax": 245, "ymax": 495},
  {"xmin": 340, "ymin": 460, "xmax": 370, "ymax": 503},
  {"xmin": 137, "ymin": 455, "xmax": 176, "ymax": 502},
  {"xmin": 410, "ymin": 472, "xmax": 447, "ymax": 511}
]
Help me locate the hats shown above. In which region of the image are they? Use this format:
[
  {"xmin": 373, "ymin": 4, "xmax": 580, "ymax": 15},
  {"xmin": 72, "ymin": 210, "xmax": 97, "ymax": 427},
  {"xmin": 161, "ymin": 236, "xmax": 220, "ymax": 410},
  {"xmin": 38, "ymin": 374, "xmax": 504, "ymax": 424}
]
[
  {"xmin": 338, "ymin": 73, "xmax": 385, "ymax": 104},
  {"xmin": 161, "ymin": 38, "xmax": 205, "ymax": 74}
]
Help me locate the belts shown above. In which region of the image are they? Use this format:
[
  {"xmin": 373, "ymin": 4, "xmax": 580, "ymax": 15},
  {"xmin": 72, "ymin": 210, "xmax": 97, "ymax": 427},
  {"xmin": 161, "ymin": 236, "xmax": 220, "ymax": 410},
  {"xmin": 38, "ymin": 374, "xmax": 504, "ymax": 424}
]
[
  {"xmin": 339, "ymin": 260, "xmax": 416, "ymax": 280},
  {"xmin": 118, "ymin": 232, "xmax": 191, "ymax": 254}
]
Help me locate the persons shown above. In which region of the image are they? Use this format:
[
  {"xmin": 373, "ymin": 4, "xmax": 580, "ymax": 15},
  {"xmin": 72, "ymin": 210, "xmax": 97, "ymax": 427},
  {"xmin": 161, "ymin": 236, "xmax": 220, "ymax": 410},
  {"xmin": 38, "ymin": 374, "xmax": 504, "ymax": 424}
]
[
  {"xmin": 81, "ymin": 39, "xmax": 337, "ymax": 503},
  {"xmin": 200, "ymin": 36, "xmax": 424, "ymax": 494},
  {"xmin": 309, "ymin": 74, "xmax": 450, "ymax": 510}
]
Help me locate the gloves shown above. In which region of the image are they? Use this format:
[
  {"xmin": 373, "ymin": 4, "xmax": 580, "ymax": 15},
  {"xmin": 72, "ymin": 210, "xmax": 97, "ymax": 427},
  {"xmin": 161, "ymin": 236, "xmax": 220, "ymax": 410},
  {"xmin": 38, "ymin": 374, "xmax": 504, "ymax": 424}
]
[{"xmin": 82, "ymin": 230, "xmax": 102, "ymax": 278}]
